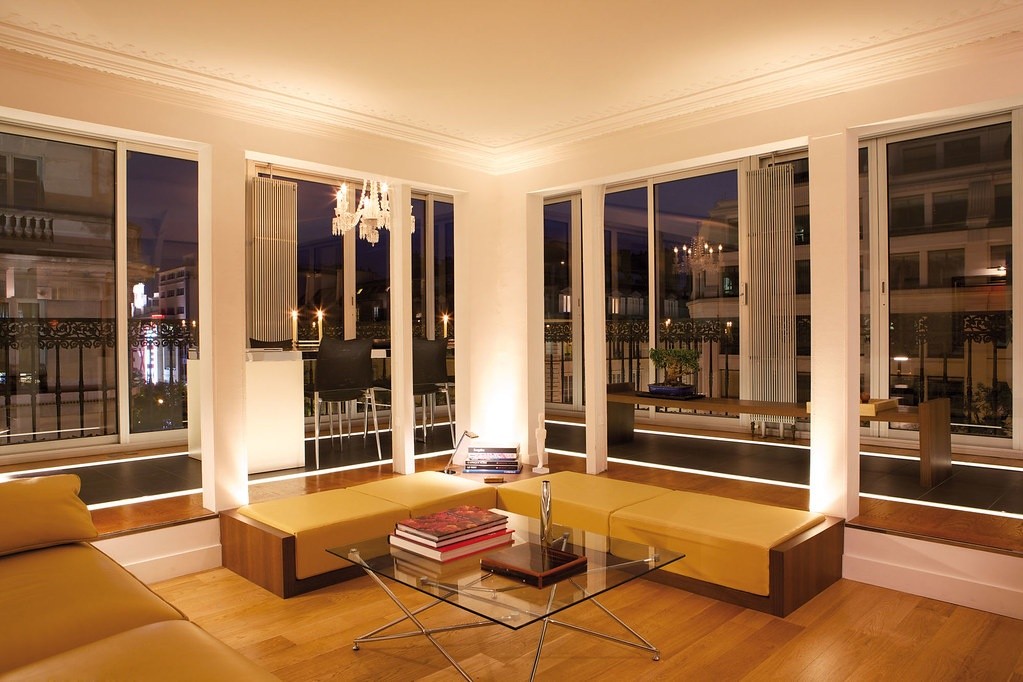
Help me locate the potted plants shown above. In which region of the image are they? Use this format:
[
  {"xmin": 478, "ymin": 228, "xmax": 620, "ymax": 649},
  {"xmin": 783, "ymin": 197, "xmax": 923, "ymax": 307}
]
[{"xmin": 647, "ymin": 347, "xmax": 702, "ymax": 396}]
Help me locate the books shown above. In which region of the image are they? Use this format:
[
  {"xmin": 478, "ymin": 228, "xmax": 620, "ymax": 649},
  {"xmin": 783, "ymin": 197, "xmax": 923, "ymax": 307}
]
[
  {"xmin": 462, "ymin": 443, "xmax": 523, "ymax": 474},
  {"xmin": 387, "ymin": 504, "xmax": 516, "ymax": 565}
]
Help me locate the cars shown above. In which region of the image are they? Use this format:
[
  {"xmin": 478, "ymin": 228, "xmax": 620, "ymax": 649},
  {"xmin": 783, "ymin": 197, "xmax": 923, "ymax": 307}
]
[
  {"xmin": 20, "ymin": 372, "xmax": 41, "ymax": 385},
  {"xmin": 0, "ymin": 373, "xmax": 6, "ymax": 384}
]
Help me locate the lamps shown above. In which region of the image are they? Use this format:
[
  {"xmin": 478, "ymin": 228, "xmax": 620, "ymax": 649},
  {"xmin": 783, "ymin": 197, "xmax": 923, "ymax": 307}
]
[
  {"xmin": 331, "ymin": 180, "xmax": 416, "ymax": 244},
  {"xmin": 671, "ymin": 224, "xmax": 726, "ymax": 276}
]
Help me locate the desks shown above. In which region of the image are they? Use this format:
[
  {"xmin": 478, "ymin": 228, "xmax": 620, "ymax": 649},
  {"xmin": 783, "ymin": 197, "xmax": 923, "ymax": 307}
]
[{"xmin": 325, "ymin": 508, "xmax": 685, "ymax": 682}]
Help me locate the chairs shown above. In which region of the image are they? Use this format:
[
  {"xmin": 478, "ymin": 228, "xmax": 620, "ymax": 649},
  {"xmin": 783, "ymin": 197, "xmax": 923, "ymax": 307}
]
[{"xmin": 303, "ymin": 337, "xmax": 456, "ymax": 470}]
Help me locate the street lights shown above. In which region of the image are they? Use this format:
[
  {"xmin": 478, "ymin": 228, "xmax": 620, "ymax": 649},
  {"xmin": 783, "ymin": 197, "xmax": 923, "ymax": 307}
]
[
  {"xmin": 443, "ymin": 314, "xmax": 448, "ymax": 340},
  {"xmin": 292, "ymin": 310, "xmax": 299, "ymax": 341},
  {"xmin": 318, "ymin": 309, "xmax": 323, "ymax": 341}
]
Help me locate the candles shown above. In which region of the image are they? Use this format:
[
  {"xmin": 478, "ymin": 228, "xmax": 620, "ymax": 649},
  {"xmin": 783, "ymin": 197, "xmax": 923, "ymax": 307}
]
[
  {"xmin": 291, "ymin": 310, "xmax": 299, "ymax": 342},
  {"xmin": 442, "ymin": 314, "xmax": 449, "ymax": 338},
  {"xmin": 317, "ymin": 310, "xmax": 323, "ymax": 341}
]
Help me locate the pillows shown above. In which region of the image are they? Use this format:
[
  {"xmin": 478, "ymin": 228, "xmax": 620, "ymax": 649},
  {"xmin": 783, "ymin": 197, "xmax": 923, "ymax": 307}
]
[{"xmin": 0, "ymin": 473, "xmax": 98, "ymax": 558}]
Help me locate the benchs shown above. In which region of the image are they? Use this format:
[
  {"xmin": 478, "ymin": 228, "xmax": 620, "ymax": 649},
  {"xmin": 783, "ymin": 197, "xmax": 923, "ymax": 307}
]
[
  {"xmin": 607, "ymin": 382, "xmax": 953, "ymax": 489},
  {"xmin": 497, "ymin": 471, "xmax": 845, "ymax": 618},
  {"xmin": 218, "ymin": 470, "xmax": 497, "ymax": 599},
  {"xmin": 0, "ymin": 540, "xmax": 284, "ymax": 682}
]
